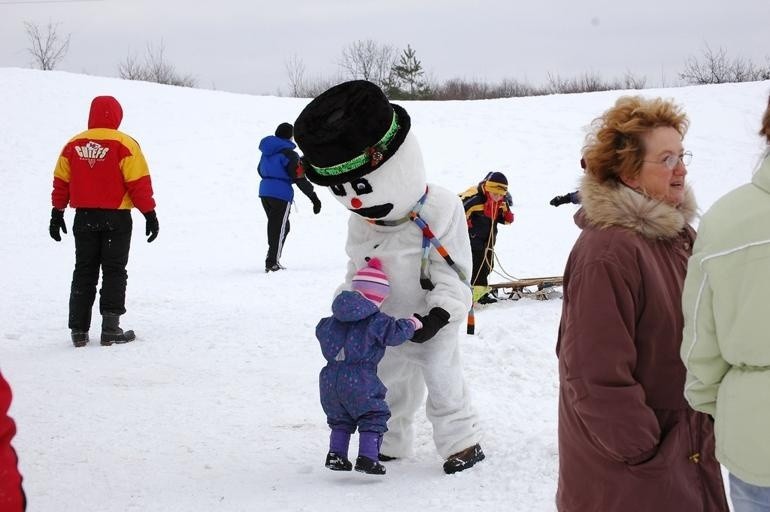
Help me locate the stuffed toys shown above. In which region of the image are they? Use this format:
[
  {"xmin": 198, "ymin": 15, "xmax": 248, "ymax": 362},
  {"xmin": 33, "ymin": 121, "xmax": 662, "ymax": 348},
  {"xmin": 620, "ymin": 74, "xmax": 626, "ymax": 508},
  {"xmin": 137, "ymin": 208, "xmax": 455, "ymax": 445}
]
[{"xmin": 292, "ymin": 79, "xmax": 488, "ymax": 473}]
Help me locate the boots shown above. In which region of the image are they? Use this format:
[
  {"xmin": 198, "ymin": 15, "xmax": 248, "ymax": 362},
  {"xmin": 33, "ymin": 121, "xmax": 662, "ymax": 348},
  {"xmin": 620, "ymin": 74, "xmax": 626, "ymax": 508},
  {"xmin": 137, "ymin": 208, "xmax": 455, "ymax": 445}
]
[
  {"xmin": 71, "ymin": 328, "xmax": 89, "ymax": 348},
  {"xmin": 101, "ymin": 310, "xmax": 136, "ymax": 347}
]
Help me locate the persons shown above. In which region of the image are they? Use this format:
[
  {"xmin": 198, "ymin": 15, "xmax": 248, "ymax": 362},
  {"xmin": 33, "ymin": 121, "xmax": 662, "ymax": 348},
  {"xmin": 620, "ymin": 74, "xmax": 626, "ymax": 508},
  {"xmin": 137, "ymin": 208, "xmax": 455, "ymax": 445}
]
[
  {"xmin": 311, "ymin": 255, "xmax": 424, "ymax": 476},
  {"xmin": 259, "ymin": 120, "xmax": 324, "ymax": 272},
  {"xmin": 1, "ymin": 369, "xmax": 28, "ymax": 511},
  {"xmin": 46, "ymin": 94, "xmax": 160, "ymax": 347},
  {"xmin": 552, "ymin": 88, "xmax": 730, "ymax": 511},
  {"xmin": 676, "ymin": 84, "xmax": 770, "ymax": 512},
  {"xmin": 550, "ymin": 153, "xmax": 589, "ymax": 207},
  {"xmin": 457, "ymin": 171, "xmax": 513, "ymax": 303}
]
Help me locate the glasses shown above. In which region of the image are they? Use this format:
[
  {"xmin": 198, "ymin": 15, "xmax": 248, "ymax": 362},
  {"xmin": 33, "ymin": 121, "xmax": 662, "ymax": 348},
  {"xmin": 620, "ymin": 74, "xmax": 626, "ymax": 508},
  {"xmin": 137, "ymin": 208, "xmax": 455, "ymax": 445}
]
[{"xmin": 641, "ymin": 151, "xmax": 693, "ymax": 170}]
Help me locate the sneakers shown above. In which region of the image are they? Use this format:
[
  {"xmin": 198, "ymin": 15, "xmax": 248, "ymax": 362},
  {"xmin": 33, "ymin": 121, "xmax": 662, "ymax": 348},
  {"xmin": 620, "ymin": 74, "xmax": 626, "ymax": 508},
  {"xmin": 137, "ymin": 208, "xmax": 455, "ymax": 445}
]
[
  {"xmin": 265, "ymin": 262, "xmax": 286, "ymax": 273},
  {"xmin": 443, "ymin": 443, "xmax": 485, "ymax": 475},
  {"xmin": 478, "ymin": 292, "xmax": 498, "ymax": 304},
  {"xmin": 355, "ymin": 455, "xmax": 386, "ymax": 475},
  {"xmin": 325, "ymin": 452, "xmax": 353, "ymax": 472},
  {"xmin": 378, "ymin": 453, "xmax": 397, "ymax": 461}
]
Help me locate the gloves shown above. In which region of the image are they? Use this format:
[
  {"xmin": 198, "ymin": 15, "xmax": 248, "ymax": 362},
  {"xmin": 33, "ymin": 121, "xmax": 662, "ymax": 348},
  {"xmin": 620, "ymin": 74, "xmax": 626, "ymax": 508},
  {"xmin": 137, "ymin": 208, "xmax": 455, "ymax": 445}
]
[
  {"xmin": 144, "ymin": 210, "xmax": 159, "ymax": 242},
  {"xmin": 407, "ymin": 316, "xmax": 424, "ymax": 331},
  {"xmin": 410, "ymin": 307, "xmax": 450, "ymax": 343},
  {"xmin": 499, "ymin": 202, "xmax": 508, "ymax": 212},
  {"xmin": 50, "ymin": 207, "xmax": 67, "ymax": 242},
  {"xmin": 309, "ymin": 192, "xmax": 322, "ymax": 214},
  {"xmin": 550, "ymin": 193, "xmax": 570, "ymax": 207}
]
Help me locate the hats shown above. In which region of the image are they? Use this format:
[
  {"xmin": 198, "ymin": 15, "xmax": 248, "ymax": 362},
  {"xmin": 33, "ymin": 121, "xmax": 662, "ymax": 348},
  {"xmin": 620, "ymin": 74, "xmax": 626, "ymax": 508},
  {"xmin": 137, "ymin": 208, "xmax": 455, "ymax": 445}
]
[
  {"xmin": 484, "ymin": 172, "xmax": 508, "ymax": 195},
  {"xmin": 293, "ymin": 80, "xmax": 411, "ymax": 186},
  {"xmin": 352, "ymin": 258, "xmax": 391, "ymax": 309},
  {"xmin": 581, "ymin": 159, "xmax": 586, "ymax": 168},
  {"xmin": 275, "ymin": 122, "xmax": 293, "ymax": 140}
]
